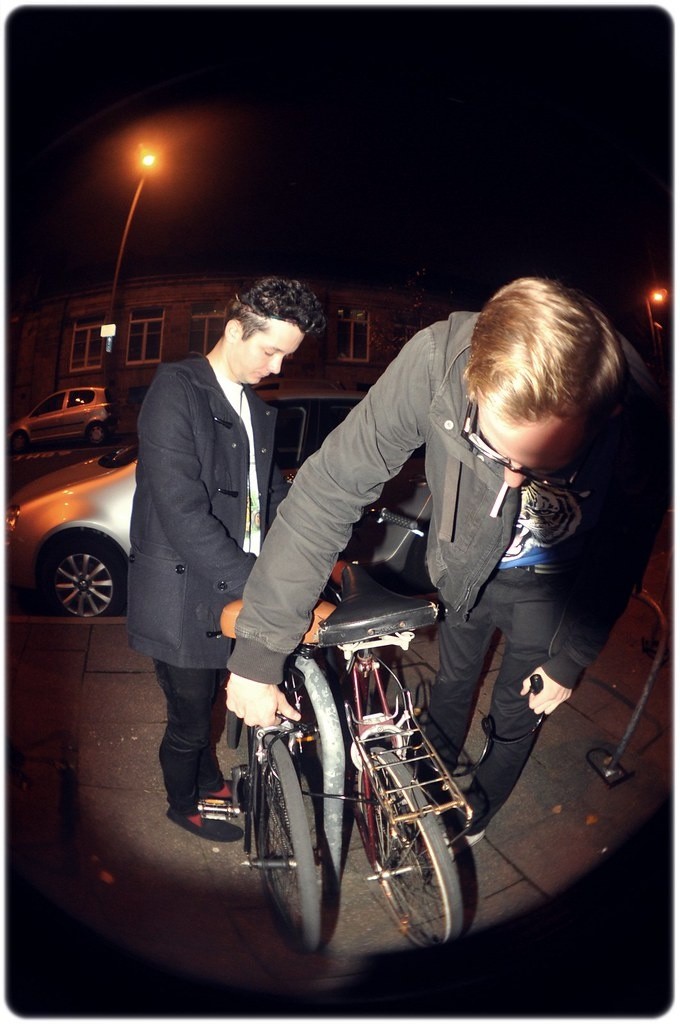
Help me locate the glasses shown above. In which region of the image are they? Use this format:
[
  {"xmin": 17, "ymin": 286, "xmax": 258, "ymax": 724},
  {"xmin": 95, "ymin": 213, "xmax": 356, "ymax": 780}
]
[{"xmin": 460, "ymin": 397, "xmax": 593, "ymax": 488}]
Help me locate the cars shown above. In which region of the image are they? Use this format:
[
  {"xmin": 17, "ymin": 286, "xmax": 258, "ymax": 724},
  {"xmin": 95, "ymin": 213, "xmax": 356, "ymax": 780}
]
[
  {"xmin": 5, "ymin": 387, "xmax": 115, "ymax": 455},
  {"xmin": 5, "ymin": 375, "xmax": 371, "ymax": 621}
]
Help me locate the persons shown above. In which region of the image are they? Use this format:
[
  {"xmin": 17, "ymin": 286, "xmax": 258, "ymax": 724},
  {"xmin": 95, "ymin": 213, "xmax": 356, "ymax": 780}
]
[
  {"xmin": 126, "ymin": 275, "xmax": 323, "ymax": 841},
  {"xmin": 223, "ymin": 276, "xmax": 675, "ymax": 864}
]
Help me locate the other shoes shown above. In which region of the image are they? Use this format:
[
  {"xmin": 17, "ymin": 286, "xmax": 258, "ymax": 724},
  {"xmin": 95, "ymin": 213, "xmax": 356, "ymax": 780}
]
[
  {"xmin": 198, "ymin": 781, "xmax": 246, "ymax": 804},
  {"xmin": 462, "ymin": 829, "xmax": 487, "ymax": 847},
  {"xmin": 167, "ymin": 804, "xmax": 244, "ymax": 843}
]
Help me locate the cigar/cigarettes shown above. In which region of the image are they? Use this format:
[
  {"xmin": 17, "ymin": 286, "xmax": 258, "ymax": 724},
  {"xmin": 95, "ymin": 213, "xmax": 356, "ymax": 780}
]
[{"xmin": 490, "ymin": 482, "xmax": 507, "ymax": 518}]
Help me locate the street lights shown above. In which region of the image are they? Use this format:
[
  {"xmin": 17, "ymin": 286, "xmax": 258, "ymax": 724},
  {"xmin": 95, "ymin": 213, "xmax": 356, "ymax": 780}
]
[
  {"xmin": 645, "ymin": 288, "xmax": 668, "ymax": 361},
  {"xmin": 100, "ymin": 154, "xmax": 159, "ymax": 386}
]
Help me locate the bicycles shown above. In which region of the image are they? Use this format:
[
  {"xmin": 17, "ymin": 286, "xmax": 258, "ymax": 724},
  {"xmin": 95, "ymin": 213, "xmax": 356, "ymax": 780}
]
[
  {"xmin": 218, "ymin": 586, "xmax": 344, "ymax": 959},
  {"xmin": 319, "ymin": 558, "xmax": 473, "ymax": 949}
]
